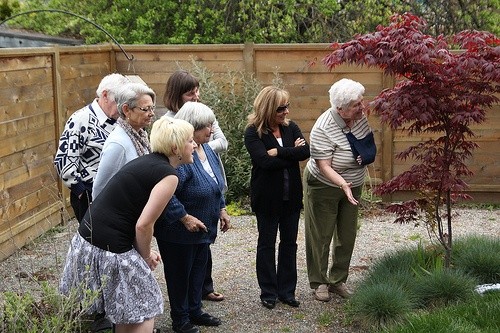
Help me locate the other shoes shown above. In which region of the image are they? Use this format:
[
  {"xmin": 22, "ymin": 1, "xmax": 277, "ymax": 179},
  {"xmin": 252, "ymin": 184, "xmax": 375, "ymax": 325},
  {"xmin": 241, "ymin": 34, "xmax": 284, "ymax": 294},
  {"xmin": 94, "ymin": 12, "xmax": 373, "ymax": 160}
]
[{"xmin": 207, "ymin": 292, "xmax": 224, "ymax": 301}]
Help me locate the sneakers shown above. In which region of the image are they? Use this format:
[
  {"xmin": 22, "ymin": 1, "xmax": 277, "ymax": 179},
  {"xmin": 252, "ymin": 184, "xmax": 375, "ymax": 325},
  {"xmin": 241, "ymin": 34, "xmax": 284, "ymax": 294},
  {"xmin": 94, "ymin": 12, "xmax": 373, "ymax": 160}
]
[
  {"xmin": 315, "ymin": 284, "xmax": 331, "ymax": 302},
  {"xmin": 329, "ymin": 283, "xmax": 353, "ymax": 299}
]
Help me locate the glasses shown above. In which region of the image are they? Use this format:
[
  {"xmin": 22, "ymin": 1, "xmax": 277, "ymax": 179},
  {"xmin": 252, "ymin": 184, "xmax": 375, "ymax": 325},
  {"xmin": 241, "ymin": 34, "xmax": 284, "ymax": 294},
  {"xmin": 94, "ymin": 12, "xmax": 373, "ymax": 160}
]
[
  {"xmin": 135, "ymin": 105, "xmax": 155, "ymax": 111},
  {"xmin": 277, "ymin": 103, "xmax": 290, "ymax": 113}
]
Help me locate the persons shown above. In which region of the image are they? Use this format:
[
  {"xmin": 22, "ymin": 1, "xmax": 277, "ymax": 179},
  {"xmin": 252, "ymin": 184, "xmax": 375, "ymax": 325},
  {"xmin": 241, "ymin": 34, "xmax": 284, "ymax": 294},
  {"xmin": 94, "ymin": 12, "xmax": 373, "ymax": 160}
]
[
  {"xmin": 244, "ymin": 85, "xmax": 311, "ymax": 309},
  {"xmin": 152, "ymin": 69, "xmax": 230, "ymax": 299},
  {"xmin": 60, "ymin": 115, "xmax": 197, "ymax": 333},
  {"xmin": 167, "ymin": 100, "xmax": 221, "ymax": 333},
  {"xmin": 304, "ymin": 78, "xmax": 375, "ymax": 303},
  {"xmin": 54, "ymin": 72, "xmax": 127, "ymax": 224},
  {"xmin": 93, "ymin": 83, "xmax": 157, "ymax": 204}
]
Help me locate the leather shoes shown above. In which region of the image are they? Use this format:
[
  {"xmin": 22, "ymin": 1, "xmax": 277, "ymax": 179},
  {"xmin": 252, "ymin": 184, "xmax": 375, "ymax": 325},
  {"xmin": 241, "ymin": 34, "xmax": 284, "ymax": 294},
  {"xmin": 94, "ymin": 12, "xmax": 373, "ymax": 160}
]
[
  {"xmin": 190, "ymin": 313, "xmax": 221, "ymax": 325},
  {"xmin": 172, "ymin": 324, "xmax": 201, "ymax": 333},
  {"xmin": 279, "ymin": 297, "xmax": 300, "ymax": 307},
  {"xmin": 262, "ymin": 299, "xmax": 276, "ymax": 308}
]
